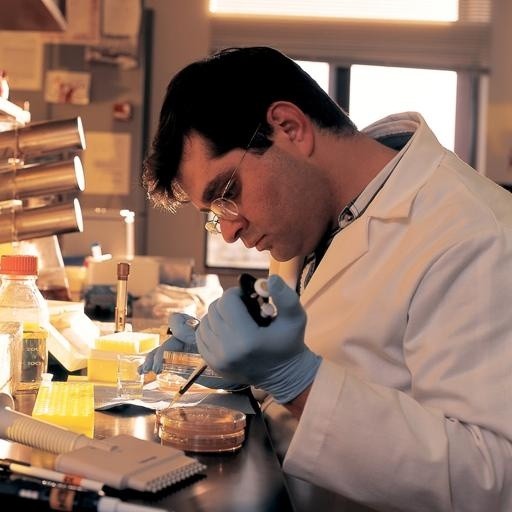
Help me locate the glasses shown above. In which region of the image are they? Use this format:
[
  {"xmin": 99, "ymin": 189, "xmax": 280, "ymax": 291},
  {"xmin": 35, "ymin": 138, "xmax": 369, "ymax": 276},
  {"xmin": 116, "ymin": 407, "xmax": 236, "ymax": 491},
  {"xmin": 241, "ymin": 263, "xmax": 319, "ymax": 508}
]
[{"xmin": 204, "ymin": 115, "xmax": 268, "ymax": 237}]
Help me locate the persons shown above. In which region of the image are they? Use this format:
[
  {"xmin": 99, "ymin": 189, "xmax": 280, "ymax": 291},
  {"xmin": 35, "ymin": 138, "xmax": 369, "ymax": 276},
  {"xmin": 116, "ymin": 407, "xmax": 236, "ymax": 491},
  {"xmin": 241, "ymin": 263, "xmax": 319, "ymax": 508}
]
[{"xmin": 135, "ymin": 44, "xmax": 512, "ymax": 512}]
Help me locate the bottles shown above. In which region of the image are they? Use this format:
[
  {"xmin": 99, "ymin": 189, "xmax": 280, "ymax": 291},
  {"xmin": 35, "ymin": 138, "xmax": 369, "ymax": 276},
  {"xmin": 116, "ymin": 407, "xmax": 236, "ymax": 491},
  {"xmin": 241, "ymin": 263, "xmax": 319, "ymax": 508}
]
[{"xmin": 1, "ymin": 255, "xmax": 52, "ymax": 394}]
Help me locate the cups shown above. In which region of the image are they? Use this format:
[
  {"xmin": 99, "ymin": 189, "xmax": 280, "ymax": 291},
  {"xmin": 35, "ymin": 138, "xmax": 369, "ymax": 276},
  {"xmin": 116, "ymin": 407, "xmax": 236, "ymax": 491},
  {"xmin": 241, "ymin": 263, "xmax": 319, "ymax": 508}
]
[
  {"xmin": 155, "ymin": 408, "xmax": 176, "ymax": 441},
  {"xmin": 116, "ymin": 355, "xmax": 147, "ymax": 398},
  {"xmin": 157, "ymin": 373, "xmax": 185, "ymax": 394},
  {"xmin": 35, "ymin": 267, "xmax": 70, "ymax": 303}
]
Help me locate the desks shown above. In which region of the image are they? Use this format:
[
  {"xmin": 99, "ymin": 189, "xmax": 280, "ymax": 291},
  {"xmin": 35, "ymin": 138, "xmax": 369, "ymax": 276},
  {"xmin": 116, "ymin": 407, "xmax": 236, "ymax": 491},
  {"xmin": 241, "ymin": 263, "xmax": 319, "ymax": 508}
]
[{"xmin": 0, "ymin": 273, "xmax": 292, "ymax": 512}]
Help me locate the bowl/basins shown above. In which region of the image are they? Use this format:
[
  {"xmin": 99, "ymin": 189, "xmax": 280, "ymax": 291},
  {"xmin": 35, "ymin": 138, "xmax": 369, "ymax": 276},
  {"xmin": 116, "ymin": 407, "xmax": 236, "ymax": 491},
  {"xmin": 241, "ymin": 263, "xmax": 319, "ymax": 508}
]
[
  {"xmin": 161, "ymin": 404, "xmax": 246, "ymax": 451},
  {"xmin": 162, "ymin": 351, "xmax": 223, "ymax": 379}
]
